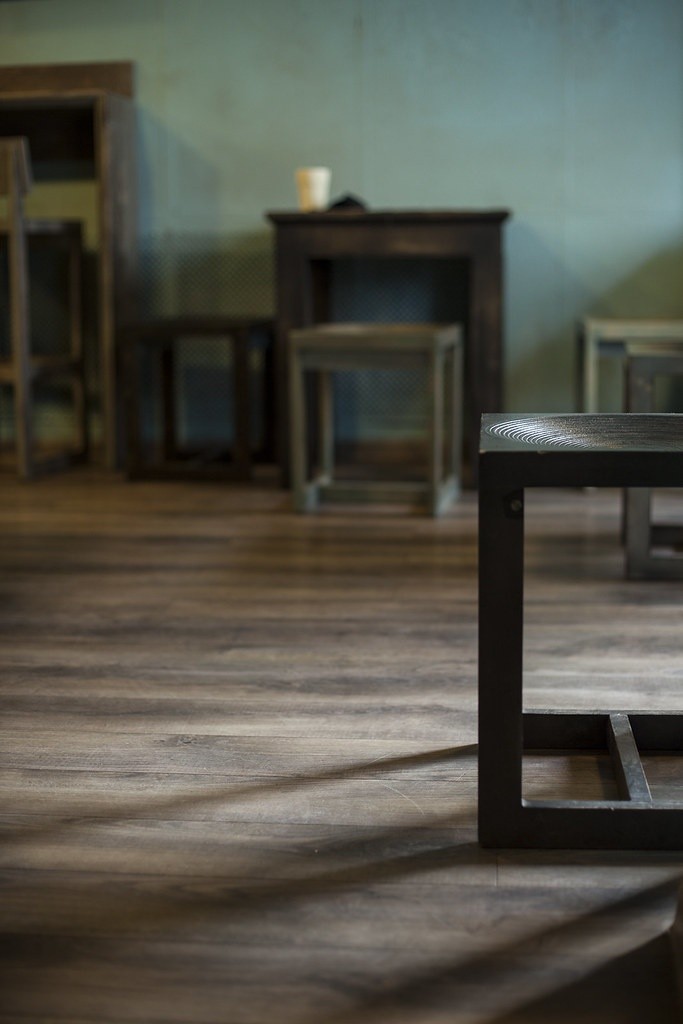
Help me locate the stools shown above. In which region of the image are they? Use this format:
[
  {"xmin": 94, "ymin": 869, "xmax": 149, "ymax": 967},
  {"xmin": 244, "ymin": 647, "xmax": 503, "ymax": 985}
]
[
  {"xmin": 286, "ymin": 321, "xmax": 464, "ymax": 518},
  {"xmin": 578, "ymin": 315, "xmax": 683, "ymax": 494},
  {"xmin": 478, "ymin": 412, "xmax": 683, "ymax": 849},
  {"xmin": 116, "ymin": 311, "xmax": 277, "ymax": 485},
  {"xmin": 619, "ymin": 338, "xmax": 683, "ymax": 583}
]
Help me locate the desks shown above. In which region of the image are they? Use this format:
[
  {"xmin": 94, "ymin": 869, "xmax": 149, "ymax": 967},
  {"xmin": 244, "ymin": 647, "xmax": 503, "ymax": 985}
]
[
  {"xmin": 262, "ymin": 205, "xmax": 517, "ymax": 494},
  {"xmin": 0, "ymin": 60, "xmax": 140, "ymax": 493}
]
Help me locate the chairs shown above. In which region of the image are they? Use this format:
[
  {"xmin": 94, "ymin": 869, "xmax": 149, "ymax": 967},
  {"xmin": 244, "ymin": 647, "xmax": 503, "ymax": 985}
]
[{"xmin": 0, "ymin": 137, "xmax": 90, "ymax": 481}]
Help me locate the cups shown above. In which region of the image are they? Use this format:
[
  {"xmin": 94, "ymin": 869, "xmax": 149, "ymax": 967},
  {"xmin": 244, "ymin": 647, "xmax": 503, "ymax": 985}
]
[{"xmin": 295, "ymin": 167, "xmax": 332, "ymax": 212}]
